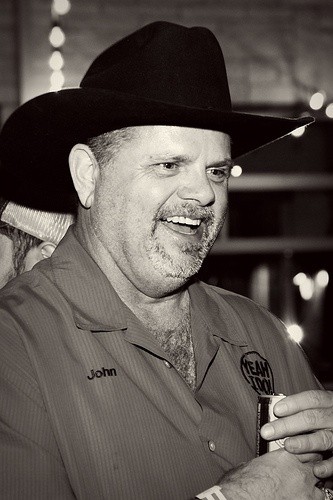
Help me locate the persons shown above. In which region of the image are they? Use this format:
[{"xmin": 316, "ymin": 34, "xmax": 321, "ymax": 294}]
[
  {"xmin": 1, "ymin": 200, "xmax": 74, "ymax": 298},
  {"xmin": 1, "ymin": 19, "xmax": 333, "ymax": 500}
]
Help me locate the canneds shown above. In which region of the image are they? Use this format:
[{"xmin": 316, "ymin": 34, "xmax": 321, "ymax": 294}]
[{"xmin": 254, "ymin": 391, "xmax": 294, "ymax": 458}]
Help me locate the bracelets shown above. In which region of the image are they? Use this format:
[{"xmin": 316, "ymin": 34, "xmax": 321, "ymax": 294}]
[{"xmin": 195, "ymin": 484, "xmax": 226, "ymax": 500}]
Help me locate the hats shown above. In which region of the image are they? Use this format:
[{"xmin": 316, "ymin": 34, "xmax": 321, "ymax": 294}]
[
  {"xmin": 1, "ymin": 19, "xmax": 316, "ymax": 221},
  {"xmin": 1, "ymin": 200, "xmax": 77, "ymax": 253}
]
